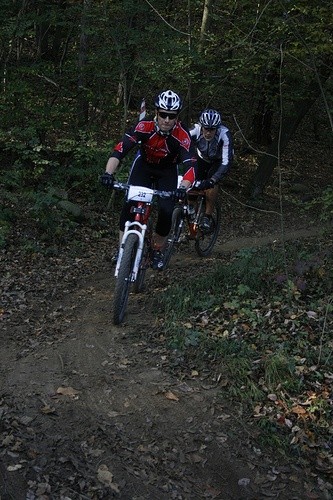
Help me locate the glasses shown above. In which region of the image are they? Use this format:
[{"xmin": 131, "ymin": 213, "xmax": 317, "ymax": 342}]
[{"xmin": 158, "ymin": 111, "xmax": 177, "ymax": 120}]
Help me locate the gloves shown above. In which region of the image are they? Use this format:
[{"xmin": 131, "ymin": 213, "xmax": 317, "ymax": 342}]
[
  {"xmin": 202, "ymin": 178, "xmax": 214, "ymax": 189},
  {"xmin": 99, "ymin": 173, "xmax": 115, "ymax": 189},
  {"xmin": 171, "ymin": 188, "xmax": 186, "ymax": 202}
]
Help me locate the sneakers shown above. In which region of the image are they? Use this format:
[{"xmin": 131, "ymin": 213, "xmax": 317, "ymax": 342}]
[
  {"xmin": 111, "ymin": 250, "xmax": 119, "ymax": 264},
  {"xmin": 200, "ymin": 216, "xmax": 210, "ymax": 233},
  {"xmin": 149, "ymin": 247, "xmax": 165, "ymax": 270}
]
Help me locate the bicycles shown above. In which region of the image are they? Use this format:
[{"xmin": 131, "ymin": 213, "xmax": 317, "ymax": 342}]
[
  {"xmin": 100, "ymin": 173, "xmax": 200, "ymax": 322},
  {"xmin": 158, "ymin": 187, "xmax": 222, "ymax": 273}
]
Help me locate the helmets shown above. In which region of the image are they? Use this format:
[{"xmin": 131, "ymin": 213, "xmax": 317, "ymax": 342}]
[
  {"xmin": 199, "ymin": 109, "xmax": 221, "ymax": 128},
  {"xmin": 154, "ymin": 90, "xmax": 182, "ymax": 113}
]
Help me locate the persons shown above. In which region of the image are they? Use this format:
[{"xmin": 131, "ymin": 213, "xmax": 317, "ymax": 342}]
[
  {"xmin": 176, "ymin": 109, "xmax": 233, "ymax": 235},
  {"xmin": 98, "ymin": 90, "xmax": 204, "ymax": 270}
]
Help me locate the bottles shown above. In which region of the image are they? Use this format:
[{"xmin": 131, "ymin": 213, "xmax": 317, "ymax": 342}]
[{"xmin": 189, "ymin": 205, "xmax": 196, "ymax": 222}]
